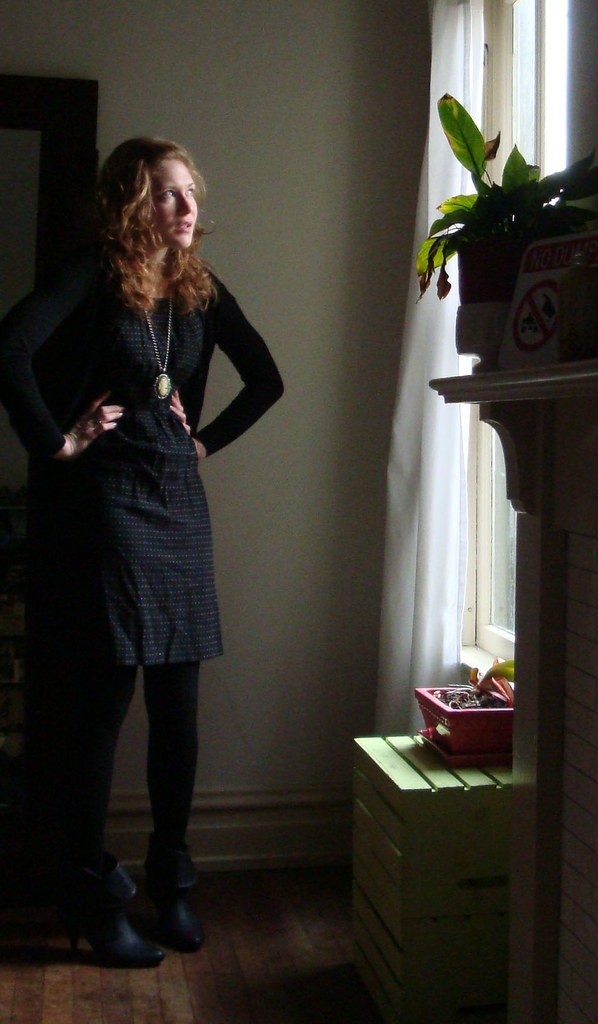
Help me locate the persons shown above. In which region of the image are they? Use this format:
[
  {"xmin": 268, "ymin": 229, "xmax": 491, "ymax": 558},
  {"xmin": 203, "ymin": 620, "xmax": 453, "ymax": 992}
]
[{"xmin": 1, "ymin": 137, "xmax": 284, "ymax": 969}]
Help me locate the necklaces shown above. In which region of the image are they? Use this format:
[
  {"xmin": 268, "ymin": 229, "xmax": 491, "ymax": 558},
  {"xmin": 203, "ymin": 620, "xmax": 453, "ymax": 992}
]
[{"xmin": 145, "ymin": 288, "xmax": 174, "ymax": 399}]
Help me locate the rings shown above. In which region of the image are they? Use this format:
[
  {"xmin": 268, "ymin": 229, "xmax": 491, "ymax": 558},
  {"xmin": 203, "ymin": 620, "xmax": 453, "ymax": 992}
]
[{"xmin": 96, "ymin": 418, "xmax": 102, "ymax": 425}]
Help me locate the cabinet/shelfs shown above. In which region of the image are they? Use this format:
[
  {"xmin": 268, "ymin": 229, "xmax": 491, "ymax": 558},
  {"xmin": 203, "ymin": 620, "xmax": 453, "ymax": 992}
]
[{"xmin": 352, "ymin": 736, "xmax": 513, "ymax": 1024}]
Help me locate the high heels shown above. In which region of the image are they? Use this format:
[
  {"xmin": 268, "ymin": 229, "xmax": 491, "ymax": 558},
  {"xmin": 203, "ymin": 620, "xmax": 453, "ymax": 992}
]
[
  {"xmin": 62, "ymin": 859, "xmax": 165, "ymax": 969},
  {"xmin": 144, "ymin": 833, "xmax": 205, "ymax": 949}
]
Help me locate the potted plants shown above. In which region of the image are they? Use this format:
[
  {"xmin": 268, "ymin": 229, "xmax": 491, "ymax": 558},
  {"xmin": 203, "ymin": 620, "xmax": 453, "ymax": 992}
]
[
  {"xmin": 415, "ymin": 94, "xmax": 598, "ymax": 375},
  {"xmin": 413, "ymin": 661, "xmax": 515, "ymax": 755}
]
